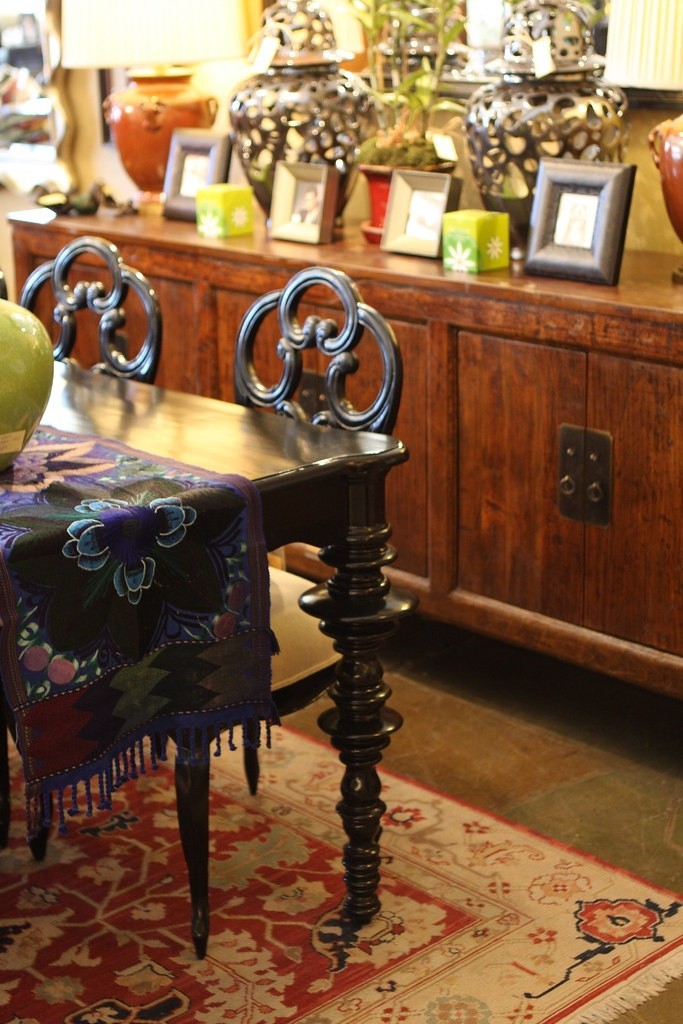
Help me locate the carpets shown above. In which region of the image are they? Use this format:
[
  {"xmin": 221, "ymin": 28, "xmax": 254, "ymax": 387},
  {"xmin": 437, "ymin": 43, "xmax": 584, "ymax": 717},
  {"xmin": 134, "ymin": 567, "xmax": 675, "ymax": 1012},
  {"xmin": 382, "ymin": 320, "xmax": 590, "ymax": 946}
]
[{"xmin": 0, "ymin": 719, "xmax": 683, "ymax": 1024}]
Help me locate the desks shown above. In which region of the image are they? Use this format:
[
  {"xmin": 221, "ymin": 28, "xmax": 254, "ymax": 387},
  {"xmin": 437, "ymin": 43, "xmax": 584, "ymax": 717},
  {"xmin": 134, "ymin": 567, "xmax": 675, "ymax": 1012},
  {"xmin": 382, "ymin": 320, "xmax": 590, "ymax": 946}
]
[
  {"xmin": 0, "ymin": 357, "xmax": 410, "ymax": 927},
  {"xmin": 8, "ymin": 190, "xmax": 683, "ymax": 698}
]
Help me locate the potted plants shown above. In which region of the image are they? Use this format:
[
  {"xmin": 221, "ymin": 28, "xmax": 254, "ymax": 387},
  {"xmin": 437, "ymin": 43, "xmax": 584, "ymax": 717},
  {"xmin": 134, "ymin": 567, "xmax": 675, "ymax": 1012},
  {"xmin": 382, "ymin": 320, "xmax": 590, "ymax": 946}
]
[{"xmin": 355, "ymin": 0, "xmax": 470, "ymax": 246}]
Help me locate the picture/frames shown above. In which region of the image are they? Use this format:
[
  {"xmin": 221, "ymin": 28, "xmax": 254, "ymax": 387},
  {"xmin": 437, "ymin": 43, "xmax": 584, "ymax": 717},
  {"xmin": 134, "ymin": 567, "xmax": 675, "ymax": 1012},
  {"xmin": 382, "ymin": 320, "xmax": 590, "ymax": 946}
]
[
  {"xmin": 159, "ymin": 125, "xmax": 233, "ymax": 221},
  {"xmin": 524, "ymin": 158, "xmax": 637, "ymax": 289},
  {"xmin": 267, "ymin": 160, "xmax": 339, "ymax": 246},
  {"xmin": 379, "ymin": 168, "xmax": 452, "ymax": 264}
]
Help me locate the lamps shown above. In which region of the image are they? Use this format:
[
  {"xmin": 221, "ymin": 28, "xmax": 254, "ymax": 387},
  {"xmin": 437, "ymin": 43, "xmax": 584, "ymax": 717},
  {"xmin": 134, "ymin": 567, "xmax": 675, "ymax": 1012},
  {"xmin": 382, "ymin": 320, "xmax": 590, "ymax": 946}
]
[
  {"xmin": 61, "ymin": 1, "xmax": 235, "ymax": 217},
  {"xmin": 597, "ymin": 0, "xmax": 683, "ymax": 285}
]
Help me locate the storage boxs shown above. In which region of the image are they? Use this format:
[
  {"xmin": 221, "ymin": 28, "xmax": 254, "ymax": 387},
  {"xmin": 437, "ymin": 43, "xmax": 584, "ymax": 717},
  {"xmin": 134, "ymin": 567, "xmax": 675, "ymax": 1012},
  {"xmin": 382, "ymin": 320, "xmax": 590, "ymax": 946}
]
[
  {"xmin": 197, "ymin": 182, "xmax": 257, "ymax": 241},
  {"xmin": 442, "ymin": 206, "xmax": 510, "ymax": 275}
]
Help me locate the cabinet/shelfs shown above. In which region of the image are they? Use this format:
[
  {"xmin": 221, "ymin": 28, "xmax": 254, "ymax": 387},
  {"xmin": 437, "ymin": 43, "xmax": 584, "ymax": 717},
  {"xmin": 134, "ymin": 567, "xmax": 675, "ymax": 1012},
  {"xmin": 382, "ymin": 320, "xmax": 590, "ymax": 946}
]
[{"xmin": 8, "ymin": 205, "xmax": 683, "ymax": 696}]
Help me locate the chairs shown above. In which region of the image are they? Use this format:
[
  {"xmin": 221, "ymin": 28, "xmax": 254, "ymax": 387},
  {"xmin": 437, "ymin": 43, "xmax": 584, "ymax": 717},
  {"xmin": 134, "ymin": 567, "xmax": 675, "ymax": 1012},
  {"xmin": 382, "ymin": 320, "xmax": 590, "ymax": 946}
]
[
  {"xmin": 26, "ymin": 265, "xmax": 402, "ymax": 962},
  {"xmin": 0, "ymin": 236, "xmax": 163, "ymax": 861}
]
